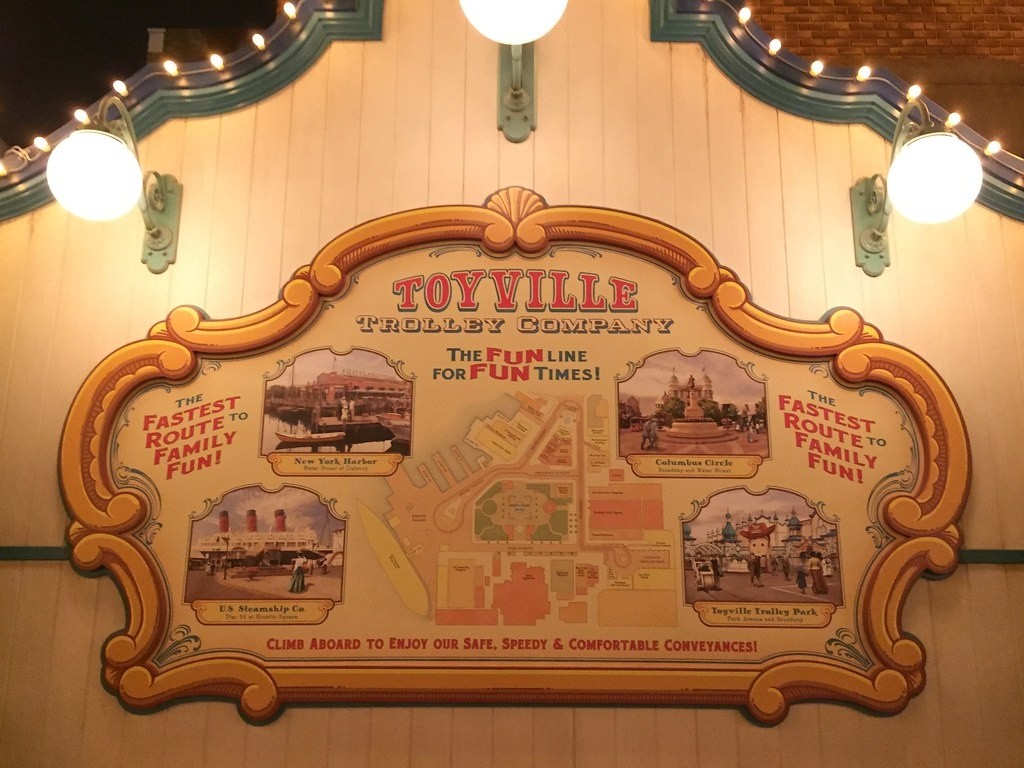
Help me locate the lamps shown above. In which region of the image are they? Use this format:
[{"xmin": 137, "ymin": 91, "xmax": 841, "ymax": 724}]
[
  {"xmin": 47, "ymin": 96, "xmax": 182, "ymax": 273},
  {"xmin": 457, "ymin": 0, "xmax": 569, "ymax": 141},
  {"xmin": 850, "ymin": 97, "xmax": 982, "ymax": 277}
]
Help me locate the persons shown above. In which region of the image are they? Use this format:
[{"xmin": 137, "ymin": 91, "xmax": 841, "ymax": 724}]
[
  {"xmin": 288, "ymin": 552, "xmax": 308, "ymax": 594},
  {"xmin": 641, "ymin": 417, "xmax": 661, "ymax": 450},
  {"xmin": 697, "ymin": 556, "xmax": 722, "ymax": 590},
  {"xmin": 742, "ymin": 417, "xmax": 767, "ymax": 443},
  {"xmin": 337, "ymin": 395, "xmax": 356, "ymax": 422},
  {"xmin": 747, "ymin": 547, "xmax": 834, "ymax": 597}
]
